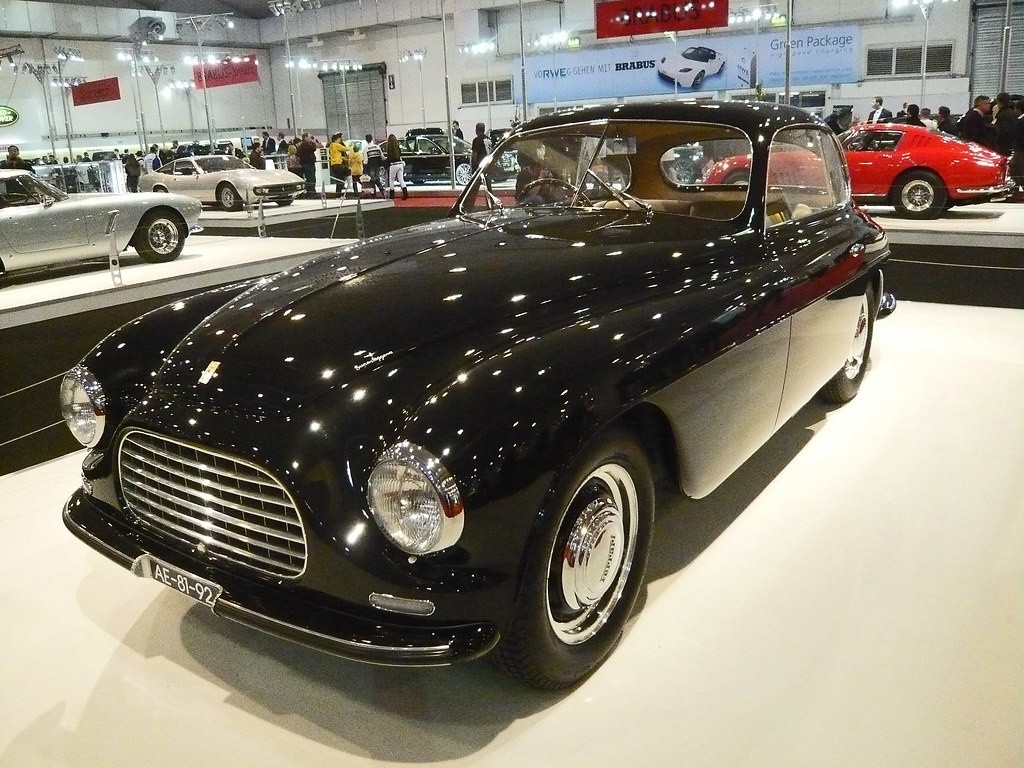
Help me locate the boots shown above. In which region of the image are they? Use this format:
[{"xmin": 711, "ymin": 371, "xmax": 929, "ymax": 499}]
[
  {"xmin": 390, "ymin": 189, "xmax": 395, "ymax": 199},
  {"xmin": 401, "ymin": 187, "xmax": 409, "ymax": 200}
]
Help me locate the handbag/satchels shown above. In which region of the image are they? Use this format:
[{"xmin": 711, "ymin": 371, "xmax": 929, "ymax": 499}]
[
  {"xmin": 359, "ymin": 172, "xmax": 372, "ymax": 182},
  {"xmin": 153, "ymin": 155, "xmax": 160, "ymax": 170}
]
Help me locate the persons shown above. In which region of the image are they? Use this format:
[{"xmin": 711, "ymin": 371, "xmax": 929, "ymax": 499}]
[
  {"xmin": 347, "ymin": 145, "xmax": 365, "ymax": 197},
  {"xmin": 362, "ymin": 133, "xmax": 389, "ymax": 200},
  {"xmin": 386, "ymin": 133, "xmax": 410, "ymax": 201},
  {"xmin": 462, "ymin": 122, "xmax": 495, "ymax": 213},
  {"xmin": 0, "ymin": 129, "xmax": 351, "ymax": 199},
  {"xmin": 452, "ymin": 120, "xmax": 464, "ymax": 154},
  {"xmin": 511, "ymin": 91, "xmax": 1024, "ymax": 211}
]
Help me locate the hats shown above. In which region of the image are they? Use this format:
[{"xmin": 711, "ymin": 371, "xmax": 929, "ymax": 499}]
[{"xmin": 293, "ymin": 138, "xmax": 302, "ymax": 144}]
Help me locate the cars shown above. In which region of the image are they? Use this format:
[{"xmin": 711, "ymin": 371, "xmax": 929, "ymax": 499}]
[
  {"xmin": 0, "ymin": 166, "xmax": 204, "ymax": 280},
  {"xmin": 57, "ymin": 94, "xmax": 898, "ymax": 698},
  {"xmin": 139, "ymin": 154, "xmax": 307, "ymax": 212},
  {"xmin": 658, "ymin": 46, "xmax": 726, "ymax": 88},
  {"xmin": 361, "ymin": 128, "xmax": 518, "ymax": 187},
  {"xmin": 700, "ymin": 118, "xmax": 1018, "ymax": 222}
]
